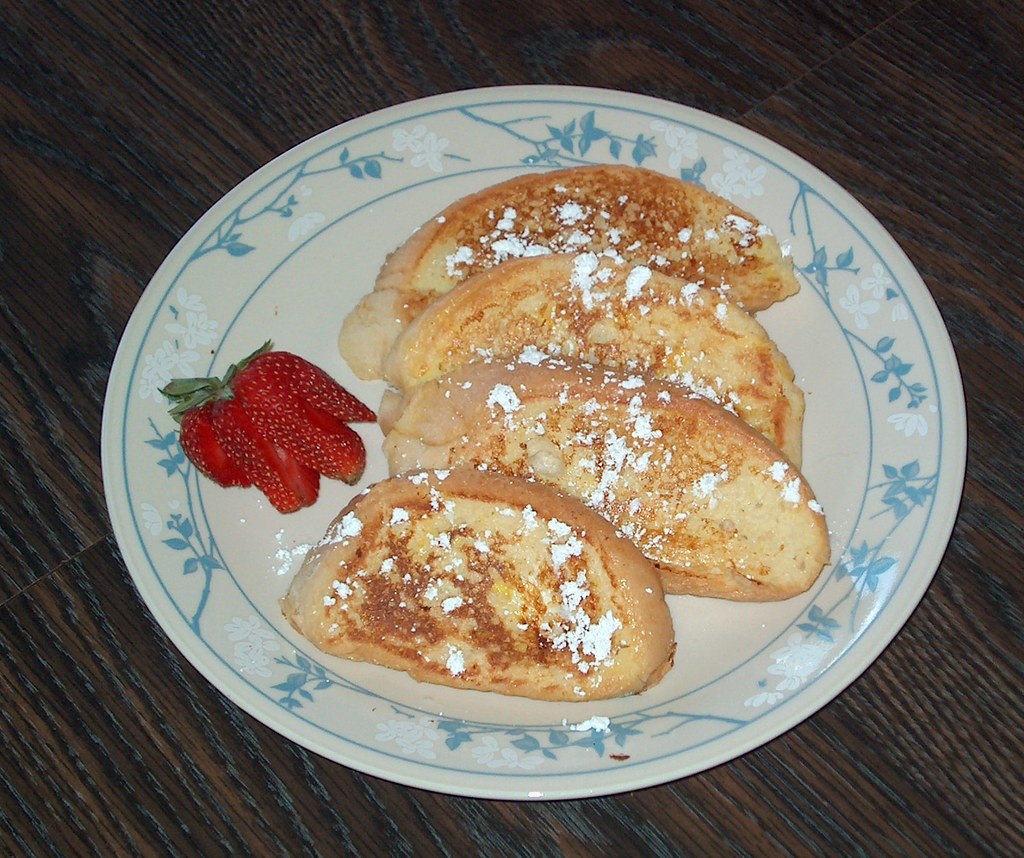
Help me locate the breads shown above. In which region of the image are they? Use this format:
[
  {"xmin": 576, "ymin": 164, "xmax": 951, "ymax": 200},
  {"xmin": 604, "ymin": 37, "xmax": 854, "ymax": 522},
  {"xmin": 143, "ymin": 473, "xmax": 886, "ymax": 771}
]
[
  {"xmin": 377, "ymin": 255, "xmax": 805, "ymax": 479},
  {"xmin": 333, "ymin": 161, "xmax": 802, "ymax": 382},
  {"xmin": 374, "ymin": 354, "xmax": 830, "ymax": 604},
  {"xmin": 280, "ymin": 467, "xmax": 676, "ymax": 704}
]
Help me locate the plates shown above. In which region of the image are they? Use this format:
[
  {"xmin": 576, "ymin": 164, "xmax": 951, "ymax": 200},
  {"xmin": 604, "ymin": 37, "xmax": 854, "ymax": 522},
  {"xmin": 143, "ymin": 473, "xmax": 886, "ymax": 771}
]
[{"xmin": 100, "ymin": 83, "xmax": 969, "ymax": 802}]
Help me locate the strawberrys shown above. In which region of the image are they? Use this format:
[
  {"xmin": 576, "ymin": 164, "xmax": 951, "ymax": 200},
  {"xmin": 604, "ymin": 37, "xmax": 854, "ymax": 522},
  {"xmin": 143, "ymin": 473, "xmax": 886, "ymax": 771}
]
[{"xmin": 156, "ymin": 340, "xmax": 379, "ymax": 515}]
